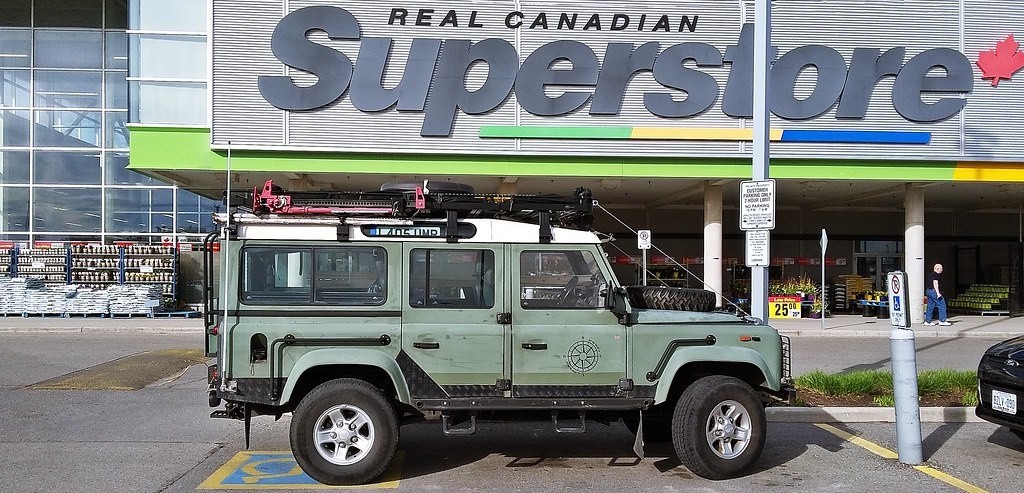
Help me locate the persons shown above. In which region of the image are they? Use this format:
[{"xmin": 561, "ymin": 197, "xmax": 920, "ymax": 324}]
[{"xmin": 924, "ymin": 263, "xmax": 952, "ymax": 326}]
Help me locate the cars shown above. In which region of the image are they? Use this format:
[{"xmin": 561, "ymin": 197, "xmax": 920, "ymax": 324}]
[{"xmin": 975, "ymin": 335, "xmax": 1024, "ymax": 432}]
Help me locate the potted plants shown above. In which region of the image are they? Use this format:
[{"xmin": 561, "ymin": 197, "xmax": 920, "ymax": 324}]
[
  {"xmin": 767, "ymin": 272, "xmax": 829, "ymax": 318},
  {"xmin": 70, "ymin": 246, "xmax": 174, "ymax": 293},
  {"xmin": 730, "ymin": 282, "xmax": 752, "ymax": 315}
]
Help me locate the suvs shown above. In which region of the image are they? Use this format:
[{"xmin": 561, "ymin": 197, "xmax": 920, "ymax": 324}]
[{"xmin": 204, "ymin": 183, "xmax": 796, "ymax": 486}]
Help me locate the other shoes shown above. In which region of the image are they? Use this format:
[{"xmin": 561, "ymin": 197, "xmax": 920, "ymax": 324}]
[
  {"xmin": 939, "ymin": 320, "xmax": 953, "ymax": 326},
  {"xmin": 924, "ymin": 321, "xmax": 936, "ymax": 326}
]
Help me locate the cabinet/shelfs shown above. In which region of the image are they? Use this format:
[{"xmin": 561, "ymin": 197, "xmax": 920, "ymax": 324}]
[
  {"xmin": 637, "ymin": 257, "xmax": 689, "ymax": 288},
  {"xmin": 0, "ymin": 244, "xmax": 181, "ymax": 301}
]
